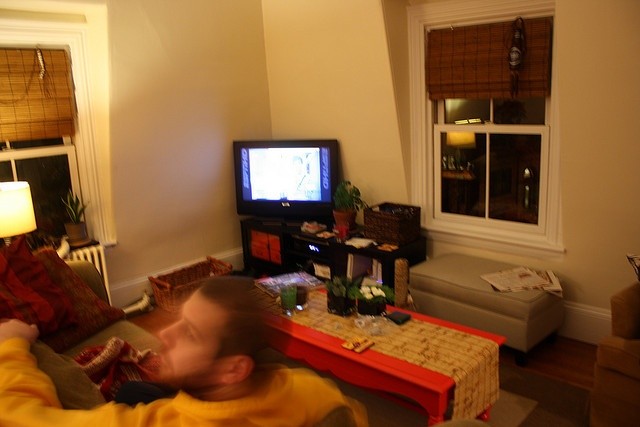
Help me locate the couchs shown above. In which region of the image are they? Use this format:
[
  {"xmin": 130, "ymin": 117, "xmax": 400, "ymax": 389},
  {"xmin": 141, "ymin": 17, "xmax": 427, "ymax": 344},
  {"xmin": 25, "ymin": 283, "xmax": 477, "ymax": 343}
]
[
  {"xmin": 596, "ymin": 280, "xmax": 640, "ymax": 378},
  {"xmin": 31, "ymin": 260, "xmax": 170, "ymax": 414}
]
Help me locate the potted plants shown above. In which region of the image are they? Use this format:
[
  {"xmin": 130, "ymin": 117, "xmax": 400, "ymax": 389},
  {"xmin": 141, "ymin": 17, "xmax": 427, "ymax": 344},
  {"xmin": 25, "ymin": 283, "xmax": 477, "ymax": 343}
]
[
  {"xmin": 333, "ymin": 181, "xmax": 364, "ymax": 229},
  {"xmin": 61, "ymin": 190, "xmax": 91, "ymax": 246}
]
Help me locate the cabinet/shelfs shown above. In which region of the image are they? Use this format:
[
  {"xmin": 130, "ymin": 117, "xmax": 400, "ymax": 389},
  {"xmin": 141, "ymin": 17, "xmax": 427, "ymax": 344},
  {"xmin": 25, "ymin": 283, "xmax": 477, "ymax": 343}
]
[{"xmin": 240, "ymin": 222, "xmax": 291, "ymax": 275}]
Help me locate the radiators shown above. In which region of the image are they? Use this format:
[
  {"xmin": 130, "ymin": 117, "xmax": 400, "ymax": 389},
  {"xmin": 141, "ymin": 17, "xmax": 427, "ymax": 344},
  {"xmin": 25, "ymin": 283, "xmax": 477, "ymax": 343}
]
[{"xmin": 61, "ymin": 245, "xmax": 151, "ymax": 318}]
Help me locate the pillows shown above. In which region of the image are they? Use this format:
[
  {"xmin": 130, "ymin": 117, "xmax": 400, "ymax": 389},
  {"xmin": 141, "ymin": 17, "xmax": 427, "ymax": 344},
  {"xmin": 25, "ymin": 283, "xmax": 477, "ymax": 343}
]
[
  {"xmin": 44, "ymin": 247, "xmax": 127, "ymax": 353},
  {"xmin": 7, "ymin": 236, "xmax": 73, "ymax": 335},
  {"xmin": 2, "ymin": 256, "xmax": 55, "ymax": 328}
]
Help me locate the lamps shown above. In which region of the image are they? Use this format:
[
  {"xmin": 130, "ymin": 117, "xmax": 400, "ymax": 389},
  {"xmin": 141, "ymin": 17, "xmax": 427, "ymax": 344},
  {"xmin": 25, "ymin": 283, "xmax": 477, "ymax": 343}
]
[
  {"xmin": 0, "ymin": 181, "xmax": 37, "ymax": 244},
  {"xmin": 446, "ymin": 127, "xmax": 476, "ymax": 168}
]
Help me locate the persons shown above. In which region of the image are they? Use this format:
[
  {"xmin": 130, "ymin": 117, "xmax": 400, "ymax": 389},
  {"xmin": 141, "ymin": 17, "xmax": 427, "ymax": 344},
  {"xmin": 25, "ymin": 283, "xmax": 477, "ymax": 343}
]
[{"xmin": 0, "ymin": 276, "xmax": 356, "ymax": 427}]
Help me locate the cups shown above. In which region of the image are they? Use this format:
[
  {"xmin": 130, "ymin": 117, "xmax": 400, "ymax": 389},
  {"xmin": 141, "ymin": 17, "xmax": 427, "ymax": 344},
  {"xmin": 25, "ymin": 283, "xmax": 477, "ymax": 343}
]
[
  {"xmin": 281, "ymin": 284, "xmax": 297, "ymax": 310},
  {"xmin": 297, "ymin": 284, "xmax": 307, "ymax": 306}
]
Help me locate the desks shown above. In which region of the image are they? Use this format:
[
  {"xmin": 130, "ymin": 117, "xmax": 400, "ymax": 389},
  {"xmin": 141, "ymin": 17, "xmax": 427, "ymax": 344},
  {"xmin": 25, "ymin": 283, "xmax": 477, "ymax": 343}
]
[
  {"xmin": 291, "ymin": 218, "xmax": 426, "ymax": 297},
  {"xmin": 243, "ymin": 276, "xmax": 507, "ymax": 427}
]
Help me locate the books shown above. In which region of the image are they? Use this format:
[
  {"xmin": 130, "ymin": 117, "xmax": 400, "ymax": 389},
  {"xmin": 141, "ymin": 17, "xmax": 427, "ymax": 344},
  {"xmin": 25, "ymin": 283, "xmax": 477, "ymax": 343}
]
[{"xmin": 255, "ymin": 271, "xmax": 325, "ymax": 298}]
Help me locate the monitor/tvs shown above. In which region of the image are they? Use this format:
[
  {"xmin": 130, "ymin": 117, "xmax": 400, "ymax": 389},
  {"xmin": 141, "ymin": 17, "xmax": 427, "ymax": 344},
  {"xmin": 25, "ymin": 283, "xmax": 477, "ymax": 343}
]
[{"xmin": 233, "ymin": 139, "xmax": 337, "ymax": 233}]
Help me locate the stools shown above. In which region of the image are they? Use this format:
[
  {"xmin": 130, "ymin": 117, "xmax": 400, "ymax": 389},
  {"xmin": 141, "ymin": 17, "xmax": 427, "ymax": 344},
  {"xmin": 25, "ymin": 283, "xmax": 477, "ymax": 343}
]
[{"xmin": 409, "ymin": 253, "xmax": 566, "ymax": 369}]
